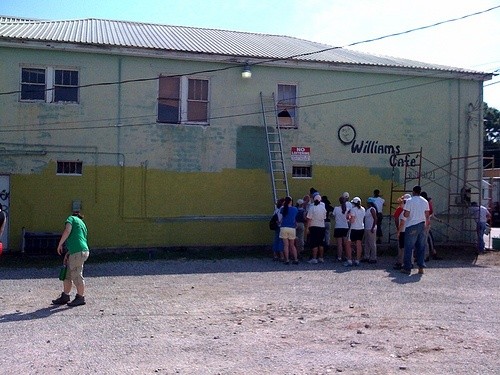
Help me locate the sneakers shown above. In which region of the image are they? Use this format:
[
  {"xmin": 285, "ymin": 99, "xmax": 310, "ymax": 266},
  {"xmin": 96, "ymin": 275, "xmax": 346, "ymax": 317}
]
[
  {"xmin": 52, "ymin": 292, "xmax": 71, "ymax": 305},
  {"xmin": 67, "ymin": 294, "xmax": 86, "ymax": 307}
]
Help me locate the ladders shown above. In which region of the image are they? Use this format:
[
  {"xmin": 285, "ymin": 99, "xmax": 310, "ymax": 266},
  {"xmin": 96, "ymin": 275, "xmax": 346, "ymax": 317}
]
[{"xmin": 260, "ymin": 91, "xmax": 293, "ymax": 208}]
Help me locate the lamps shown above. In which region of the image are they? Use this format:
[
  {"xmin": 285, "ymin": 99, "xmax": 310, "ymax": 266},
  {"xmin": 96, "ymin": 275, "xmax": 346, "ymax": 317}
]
[{"xmin": 241, "ymin": 66, "xmax": 252, "ymax": 79}]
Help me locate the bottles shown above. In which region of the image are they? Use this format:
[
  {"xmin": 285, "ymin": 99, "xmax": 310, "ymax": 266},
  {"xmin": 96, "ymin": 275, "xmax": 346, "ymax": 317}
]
[{"xmin": 59, "ymin": 264, "xmax": 67, "ymax": 281}]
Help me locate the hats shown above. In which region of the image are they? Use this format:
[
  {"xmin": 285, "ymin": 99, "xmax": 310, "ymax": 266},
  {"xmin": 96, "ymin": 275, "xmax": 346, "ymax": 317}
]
[
  {"xmin": 403, "ymin": 194, "xmax": 411, "ymax": 201},
  {"xmin": 312, "ymin": 192, "xmax": 319, "ymax": 197},
  {"xmin": 366, "ymin": 197, "xmax": 376, "ymax": 203},
  {"xmin": 296, "ymin": 199, "xmax": 305, "ymax": 205},
  {"xmin": 342, "ymin": 192, "xmax": 350, "ymax": 198},
  {"xmin": 314, "ymin": 195, "xmax": 322, "ymax": 202},
  {"xmin": 351, "ymin": 196, "xmax": 361, "ymax": 203}
]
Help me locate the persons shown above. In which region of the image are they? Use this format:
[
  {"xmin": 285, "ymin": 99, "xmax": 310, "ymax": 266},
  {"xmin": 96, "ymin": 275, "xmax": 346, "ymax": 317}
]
[
  {"xmin": 52, "ymin": 210, "xmax": 90, "ymax": 307},
  {"xmin": 469, "ymin": 202, "xmax": 491, "ymax": 252},
  {"xmin": 269, "ymin": 185, "xmax": 443, "ymax": 275}
]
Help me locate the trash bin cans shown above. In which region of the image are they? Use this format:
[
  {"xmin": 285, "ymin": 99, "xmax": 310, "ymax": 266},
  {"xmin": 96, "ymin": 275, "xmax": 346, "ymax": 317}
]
[{"xmin": 492, "ymin": 238, "xmax": 500, "ymax": 251}]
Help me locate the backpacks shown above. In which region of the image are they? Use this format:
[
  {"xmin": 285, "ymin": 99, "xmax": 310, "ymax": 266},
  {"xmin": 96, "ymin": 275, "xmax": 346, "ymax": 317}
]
[{"xmin": 269, "ymin": 210, "xmax": 280, "ymax": 231}]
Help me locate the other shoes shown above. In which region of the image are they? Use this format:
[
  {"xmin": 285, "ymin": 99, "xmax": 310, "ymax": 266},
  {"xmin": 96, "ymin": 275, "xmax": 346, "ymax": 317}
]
[
  {"xmin": 316, "ymin": 257, "xmax": 324, "ymax": 263},
  {"xmin": 393, "ymin": 265, "xmax": 403, "ymax": 270},
  {"xmin": 399, "ymin": 268, "xmax": 411, "ymax": 274},
  {"xmin": 335, "ymin": 238, "xmax": 382, "ymax": 267},
  {"xmin": 418, "ymin": 269, "xmax": 425, "ymax": 274},
  {"xmin": 432, "ymin": 253, "xmax": 443, "ymax": 260},
  {"xmin": 306, "ymin": 257, "xmax": 319, "ymax": 264},
  {"xmin": 274, "ymin": 255, "xmax": 302, "ymax": 265}
]
